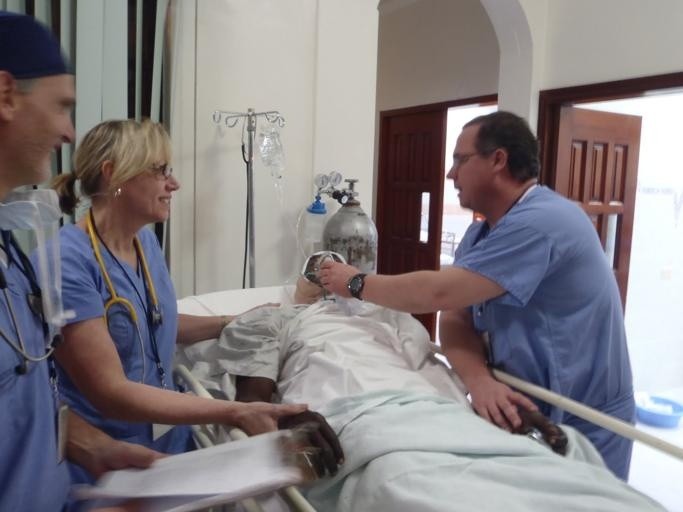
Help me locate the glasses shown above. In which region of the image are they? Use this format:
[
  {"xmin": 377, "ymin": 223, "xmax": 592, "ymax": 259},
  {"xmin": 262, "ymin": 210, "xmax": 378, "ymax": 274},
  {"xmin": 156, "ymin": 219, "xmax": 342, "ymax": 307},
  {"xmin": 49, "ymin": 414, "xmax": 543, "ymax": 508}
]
[
  {"xmin": 160, "ymin": 162, "xmax": 173, "ymax": 178},
  {"xmin": 453, "ymin": 151, "xmax": 479, "ymax": 162}
]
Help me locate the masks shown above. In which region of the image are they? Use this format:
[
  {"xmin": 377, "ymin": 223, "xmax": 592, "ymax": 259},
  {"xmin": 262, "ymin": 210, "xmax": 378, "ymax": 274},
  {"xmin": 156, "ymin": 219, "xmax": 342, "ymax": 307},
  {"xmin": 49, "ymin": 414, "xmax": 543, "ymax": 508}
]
[{"xmin": 0, "ymin": 189, "xmax": 61, "ymax": 231}]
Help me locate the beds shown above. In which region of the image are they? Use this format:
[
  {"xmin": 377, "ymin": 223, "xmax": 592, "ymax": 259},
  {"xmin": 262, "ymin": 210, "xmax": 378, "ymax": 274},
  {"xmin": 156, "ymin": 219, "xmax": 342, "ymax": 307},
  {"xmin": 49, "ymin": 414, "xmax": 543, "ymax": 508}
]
[{"xmin": 168, "ymin": 284, "xmax": 682, "ymax": 512}]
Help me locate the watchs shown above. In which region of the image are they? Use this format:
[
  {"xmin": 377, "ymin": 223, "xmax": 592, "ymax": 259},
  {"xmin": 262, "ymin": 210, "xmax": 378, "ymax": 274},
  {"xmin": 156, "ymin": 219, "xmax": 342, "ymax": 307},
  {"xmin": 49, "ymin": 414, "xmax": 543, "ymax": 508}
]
[{"xmin": 346, "ymin": 271, "xmax": 368, "ymax": 302}]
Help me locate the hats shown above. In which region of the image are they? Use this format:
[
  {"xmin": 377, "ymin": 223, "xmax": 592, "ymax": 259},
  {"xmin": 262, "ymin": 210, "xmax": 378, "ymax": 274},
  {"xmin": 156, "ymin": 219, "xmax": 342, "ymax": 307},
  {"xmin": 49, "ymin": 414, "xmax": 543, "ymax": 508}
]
[{"xmin": 0, "ymin": 11, "xmax": 76, "ymax": 80}]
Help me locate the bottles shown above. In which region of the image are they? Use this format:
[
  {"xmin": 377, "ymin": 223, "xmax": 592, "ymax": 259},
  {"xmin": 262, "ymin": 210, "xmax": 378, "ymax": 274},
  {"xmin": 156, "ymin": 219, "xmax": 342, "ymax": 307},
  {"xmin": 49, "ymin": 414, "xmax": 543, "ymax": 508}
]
[{"xmin": 305, "ymin": 202, "xmax": 326, "ymax": 242}]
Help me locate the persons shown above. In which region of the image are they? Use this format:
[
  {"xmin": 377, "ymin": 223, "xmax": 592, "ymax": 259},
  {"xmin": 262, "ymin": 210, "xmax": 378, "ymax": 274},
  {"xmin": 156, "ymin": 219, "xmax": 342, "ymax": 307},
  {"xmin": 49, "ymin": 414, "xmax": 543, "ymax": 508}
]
[
  {"xmin": 0, "ymin": 10, "xmax": 173, "ymax": 512},
  {"xmin": 31, "ymin": 114, "xmax": 308, "ymax": 437},
  {"xmin": 231, "ymin": 248, "xmax": 569, "ymax": 478},
  {"xmin": 319, "ymin": 110, "xmax": 635, "ymax": 486}
]
